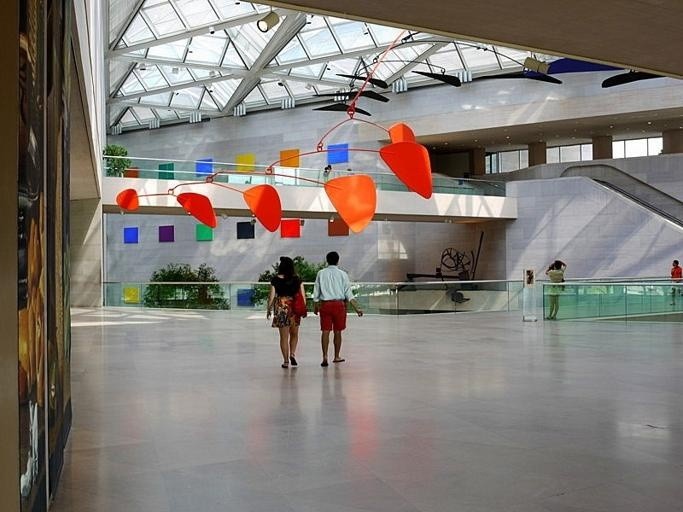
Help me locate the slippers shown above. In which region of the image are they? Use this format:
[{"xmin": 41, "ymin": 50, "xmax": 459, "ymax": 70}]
[
  {"xmin": 321, "ymin": 363, "xmax": 328, "ymax": 366},
  {"xmin": 333, "ymin": 358, "xmax": 345, "ymax": 362}
]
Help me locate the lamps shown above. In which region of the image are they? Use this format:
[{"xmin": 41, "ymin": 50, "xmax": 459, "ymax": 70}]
[
  {"xmin": 522, "ymin": 52, "xmax": 552, "ymax": 74},
  {"xmin": 257, "ymin": 6, "xmax": 279, "ymax": 33}
]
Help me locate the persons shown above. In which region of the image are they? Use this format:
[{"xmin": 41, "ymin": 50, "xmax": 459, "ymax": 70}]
[
  {"xmin": 319, "ymin": 358, "xmax": 346, "ymax": 424},
  {"xmin": 669, "ymin": 260, "xmax": 683, "ymax": 305},
  {"xmin": 278, "ymin": 367, "xmax": 299, "ymax": 408},
  {"xmin": 312, "ymin": 251, "xmax": 364, "ymax": 367},
  {"xmin": 544, "ymin": 260, "xmax": 568, "ymax": 320},
  {"xmin": 267, "ymin": 257, "xmax": 308, "ymax": 369}
]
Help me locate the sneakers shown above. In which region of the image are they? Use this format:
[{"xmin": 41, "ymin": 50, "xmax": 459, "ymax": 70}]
[{"xmin": 544, "ymin": 316, "xmax": 556, "ymax": 320}]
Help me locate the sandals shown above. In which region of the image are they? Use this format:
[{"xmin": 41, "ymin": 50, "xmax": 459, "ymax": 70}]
[
  {"xmin": 290, "ymin": 353, "xmax": 297, "ymax": 365},
  {"xmin": 282, "ymin": 362, "xmax": 289, "ymax": 368}
]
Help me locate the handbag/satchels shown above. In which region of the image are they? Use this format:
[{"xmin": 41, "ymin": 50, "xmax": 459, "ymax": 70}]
[
  {"xmin": 561, "ymin": 279, "xmax": 565, "ymax": 291},
  {"xmin": 292, "ymin": 290, "xmax": 307, "ymax": 317}
]
